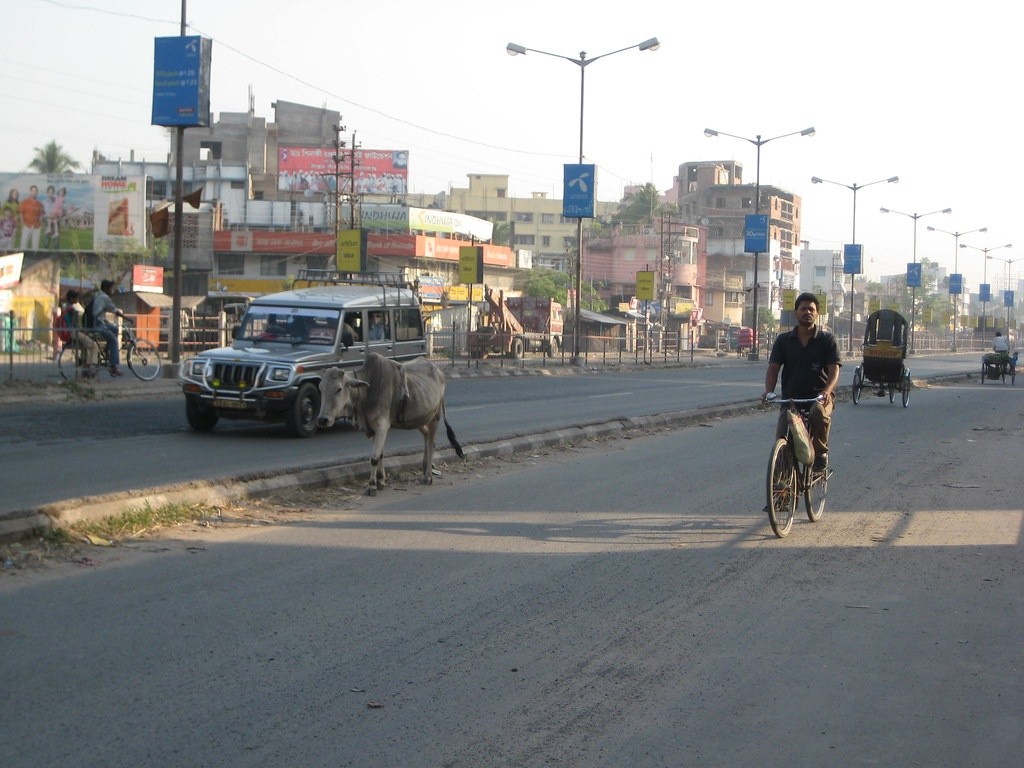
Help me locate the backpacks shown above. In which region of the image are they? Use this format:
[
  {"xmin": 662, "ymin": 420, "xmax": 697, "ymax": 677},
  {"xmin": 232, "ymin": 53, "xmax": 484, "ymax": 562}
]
[
  {"xmin": 53, "ymin": 304, "xmax": 74, "ymax": 342},
  {"xmin": 81, "ymin": 291, "xmax": 102, "ymax": 329}
]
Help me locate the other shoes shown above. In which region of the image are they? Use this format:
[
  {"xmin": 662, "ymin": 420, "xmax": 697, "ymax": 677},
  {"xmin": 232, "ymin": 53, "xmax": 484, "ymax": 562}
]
[
  {"xmin": 812, "ymin": 452, "xmax": 828, "ymax": 472},
  {"xmin": 109, "ymin": 369, "xmax": 124, "ymax": 376},
  {"xmin": 779, "ymin": 496, "xmax": 791, "ymax": 511}
]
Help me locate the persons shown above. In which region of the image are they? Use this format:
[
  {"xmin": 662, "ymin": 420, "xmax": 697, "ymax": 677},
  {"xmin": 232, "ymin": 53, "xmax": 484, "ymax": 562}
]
[
  {"xmin": 91, "ymin": 279, "xmax": 134, "ymax": 377},
  {"xmin": 62, "ymin": 292, "xmax": 98, "ymax": 376},
  {"xmin": 326, "ymin": 317, "xmax": 359, "ymax": 341},
  {"xmin": 279, "ymin": 169, "xmax": 408, "ymax": 194},
  {"xmin": 393, "ymin": 152, "xmax": 406, "ymax": 168},
  {"xmin": 993, "ymin": 332, "xmax": 1010, "ymax": 353},
  {"xmin": 367, "ymin": 312, "xmax": 386, "ymax": 341},
  {"xmin": 0, "ymin": 185, "xmax": 67, "ymax": 250},
  {"xmin": 756, "ymin": 291, "xmax": 842, "ymax": 512}
]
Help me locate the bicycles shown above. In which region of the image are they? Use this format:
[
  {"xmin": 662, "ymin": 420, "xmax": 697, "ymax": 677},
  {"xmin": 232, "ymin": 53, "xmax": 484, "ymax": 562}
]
[
  {"xmin": 57, "ymin": 322, "xmax": 162, "ymax": 381},
  {"xmin": 763, "ymin": 393, "xmax": 835, "ymax": 540}
]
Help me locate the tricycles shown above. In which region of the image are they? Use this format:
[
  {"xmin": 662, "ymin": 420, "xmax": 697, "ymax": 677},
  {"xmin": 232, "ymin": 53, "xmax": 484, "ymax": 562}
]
[
  {"xmin": 981, "ymin": 350, "xmax": 1017, "ymax": 386},
  {"xmin": 851, "ymin": 309, "xmax": 913, "ymax": 408}
]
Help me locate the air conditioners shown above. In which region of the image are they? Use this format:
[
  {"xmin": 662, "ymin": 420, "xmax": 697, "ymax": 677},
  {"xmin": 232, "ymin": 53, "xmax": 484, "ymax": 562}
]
[{"xmin": 643, "ymin": 228, "xmax": 653, "ymax": 234}]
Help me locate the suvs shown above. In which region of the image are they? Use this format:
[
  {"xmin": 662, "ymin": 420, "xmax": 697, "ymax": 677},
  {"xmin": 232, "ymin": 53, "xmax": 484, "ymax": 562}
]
[{"xmin": 178, "ymin": 269, "xmax": 426, "ymax": 439}]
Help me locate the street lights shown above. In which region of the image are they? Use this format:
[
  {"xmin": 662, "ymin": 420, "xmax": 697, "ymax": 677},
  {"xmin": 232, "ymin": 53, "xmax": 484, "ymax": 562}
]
[
  {"xmin": 705, "ymin": 127, "xmax": 817, "ymax": 360},
  {"xmin": 927, "ymin": 225, "xmax": 987, "ymax": 353},
  {"xmin": 811, "ymin": 175, "xmax": 900, "ymax": 358},
  {"xmin": 959, "ymin": 243, "xmax": 1012, "ymax": 352},
  {"xmin": 987, "ymin": 255, "xmax": 1011, "ymax": 351},
  {"xmin": 507, "ymin": 36, "xmax": 662, "ymax": 368},
  {"xmin": 880, "ymin": 206, "xmax": 952, "ymax": 354}
]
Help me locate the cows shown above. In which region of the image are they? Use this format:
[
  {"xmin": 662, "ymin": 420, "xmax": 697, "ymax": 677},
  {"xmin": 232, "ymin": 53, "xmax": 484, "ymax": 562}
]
[{"xmin": 315, "ymin": 352, "xmax": 465, "ymax": 497}]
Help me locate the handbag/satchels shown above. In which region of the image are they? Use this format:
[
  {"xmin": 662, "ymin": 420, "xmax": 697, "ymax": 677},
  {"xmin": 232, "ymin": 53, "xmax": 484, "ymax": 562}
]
[{"xmin": 786, "ymin": 397, "xmax": 816, "ymax": 466}]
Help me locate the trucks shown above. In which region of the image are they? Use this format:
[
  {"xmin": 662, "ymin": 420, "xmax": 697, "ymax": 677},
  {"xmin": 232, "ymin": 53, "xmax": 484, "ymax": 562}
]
[{"xmin": 468, "ymin": 284, "xmax": 564, "ymax": 360}]
